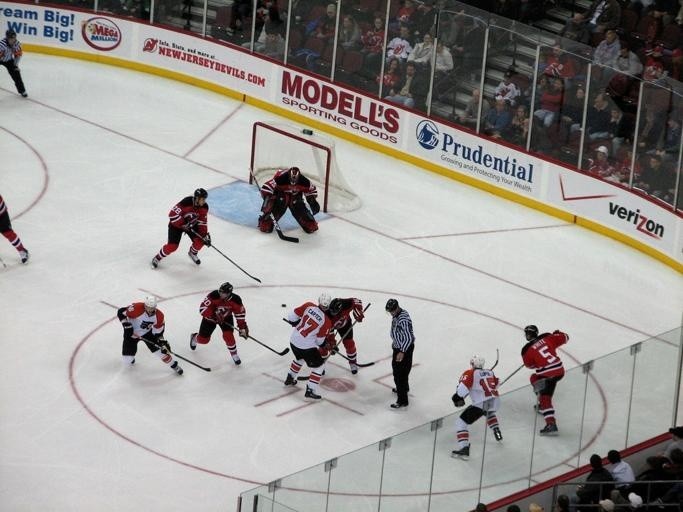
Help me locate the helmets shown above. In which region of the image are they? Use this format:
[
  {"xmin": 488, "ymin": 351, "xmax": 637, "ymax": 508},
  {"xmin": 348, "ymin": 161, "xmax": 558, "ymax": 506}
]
[
  {"xmin": 288, "ymin": 167, "xmax": 300, "ymax": 185},
  {"xmin": 194, "ymin": 188, "xmax": 208, "ymax": 198},
  {"xmin": 386, "ymin": 299, "xmax": 399, "ymax": 317},
  {"xmin": 329, "ymin": 298, "xmax": 343, "ymax": 317},
  {"xmin": 6, "ymin": 30, "xmax": 16, "ymax": 38},
  {"xmin": 469, "ymin": 354, "xmax": 485, "ymax": 369},
  {"xmin": 218, "ymin": 282, "xmax": 234, "ymax": 300},
  {"xmin": 144, "ymin": 295, "xmax": 156, "ymax": 316},
  {"xmin": 523, "ymin": 324, "xmax": 538, "ymax": 336},
  {"xmin": 318, "ymin": 293, "xmax": 331, "ymax": 312}
]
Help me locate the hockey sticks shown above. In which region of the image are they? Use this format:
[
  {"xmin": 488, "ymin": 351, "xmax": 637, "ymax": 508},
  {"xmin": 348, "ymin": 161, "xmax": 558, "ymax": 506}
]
[
  {"xmin": 284, "ymin": 318, "xmax": 374, "ymax": 367},
  {"xmin": 133, "ymin": 333, "xmax": 223, "ymax": 372},
  {"xmin": 224, "ymin": 320, "xmax": 289, "ymax": 356},
  {"xmin": 463, "ymin": 348, "xmax": 499, "ymax": 398},
  {"xmin": 248, "ymin": 166, "xmax": 299, "ymax": 243},
  {"xmin": 191, "ymin": 228, "xmax": 261, "ymax": 283},
  {"xmin": 296, "ymin": 304, "xmax": 372, "ymax": 381}
]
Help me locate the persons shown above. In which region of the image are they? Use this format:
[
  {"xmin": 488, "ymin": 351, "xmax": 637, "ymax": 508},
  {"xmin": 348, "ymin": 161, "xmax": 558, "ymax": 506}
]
[
  {"xmin": 557, "ymin": 427, "xmax": 682, "ymax": 512},
  {"xmin": 284, "ymin": 294, "xmax": 364, "ymax": 399},
  {"xmin": 190, "ymin": 282, "xmax": 249, "ymax": 365},
  {"xmin": 0, "ymin": 194, "xmax": 30, "ymax": 265},
  {"xmin": 0, "ymin": 28, "xmax": 28, "ymax": 97},
  {"xmin": 102, "ymin": 1, "xmax": 152, "ymax": 21},
  {"xmin": 385, "ymin": 298, "xmax": 415, "ymax": 408},
  {"xmin": 257, "ymin": 167, "xmax": 320, "ymax": 233},
  {"xmin": 229, "ymin": 2, "xmax": 563, "ymax": 110},
  {"xmin": 448, "ymin": 2, "xmax": 681, "ymax": 209},
  {"xmin": 475, "ymin": 503, "xmax": 544, "ymax": 512},
  {"xmin": 151, "ymin": 188, "xmax": 211, "ymax": 267},
  {"xmin": 451, "ymin": 354, "xmax": 504, "ymax": 460},
  {"xmin": 521, "ymin": 325, "xmax": 569, "ymax": 436},
  {"xmin": 117, "ymin": 297, "xmax": 183, "ymax": 375}
]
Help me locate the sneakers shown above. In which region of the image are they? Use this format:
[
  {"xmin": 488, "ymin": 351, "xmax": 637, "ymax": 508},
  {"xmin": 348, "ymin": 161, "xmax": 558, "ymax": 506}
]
[
  {"xmin": 188, "ymin": 251, "xmax": 201, "ymax": 264},
  {"xmin": 20, "ymin": 248, "xmax": 30, "ymax": 263},
  {"xmin": 190, "ymin": 333, "xmax": 197, "ymax": 351},
  {"xmin": 232, "ymin": 355, "xmax": 241, "ymax": 364},
  {"xmin": 391, "ymin": 402, "xmax": 408, "ymax": 409},
  {"xmin": 349, "ymin": 365, "xmax": 358, "ymax": 374},
  {"xmin": 171, "ymin": 361, "xmax": 183, "ymax": 375},
  {"xmin": 152, "ymin": 256, "xmax": 160, "ymax": 268},
  {"xmin": 392, "ymin": 387, "xmax": 398, "ymax": 394},
  {"xmin": 21, "ymin": 91, "xmax": 27, "ymax": 97},
  {"xmin": 540, "ymin": 423, "xmax": 558, "ymax": 434}
]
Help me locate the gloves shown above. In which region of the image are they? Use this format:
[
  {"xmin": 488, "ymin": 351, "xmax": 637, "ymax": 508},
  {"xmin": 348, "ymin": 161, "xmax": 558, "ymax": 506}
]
[
  {"xmin": 353, "ymin": 308, "xmax": 365, "ymax": 322},
  {"xmin": 201, "ymin": 233, "xmax": 212, "ymax": 248},
  {"xmin": 451, "ymin": 394, "xmax": 466, "ymax": 407},
  {"xmin": 159, "ymin": 340, "xmax": 171, "ymax": 354},
  {"xmin": 320, "ymin": 344, "xmax": 332, "ymax": 359},
  {"xmin": 239, "ymin": 326, "xmax": 249, "ymax": 340},
  {"xmin": 123, "ymin": 322, "xmax": 134, "ymax": 337},
  {"xmin": 183, "ymin": 221, "xmax": 193, "ymax": 235},
  {"xmin": 214, "ymin": 313, "xmax": 224, "ymax": 325}
]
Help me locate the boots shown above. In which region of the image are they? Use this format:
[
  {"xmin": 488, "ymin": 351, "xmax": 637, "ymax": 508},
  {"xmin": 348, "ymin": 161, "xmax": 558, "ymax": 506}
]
[
  {"xmin": 493, "ymin": 427, "xmax": 502, "ymax": 441},
  {"xmin": 305, "ymin": 384, "xmax": 322, "ymax": 399},
  {"xmin": 451, "ymin": 444, "xmax": 470, "ymax": 456},
  {"xmin": 285, "ymin": 373, "xmax": 297, "ymax": 386}
]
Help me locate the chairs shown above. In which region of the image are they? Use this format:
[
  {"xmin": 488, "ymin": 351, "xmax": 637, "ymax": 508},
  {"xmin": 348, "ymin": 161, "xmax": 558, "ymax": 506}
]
[
  {"xmin": 546, "ymin": 7, "xmax": 681, "ymax": 173},
  {"xmin": 212, "ymin": 0, "xmax": 464, "ymax": 74}
]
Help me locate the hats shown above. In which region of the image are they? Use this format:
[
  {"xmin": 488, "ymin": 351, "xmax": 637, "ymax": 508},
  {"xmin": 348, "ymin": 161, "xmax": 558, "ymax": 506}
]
[
  {"xmin": 599, "ymin": 499, "xmax": 615, "ymax": 512},
  {"xmin": 628, "ymin": 492, "xmax": 643, "ymax": 509}
]
